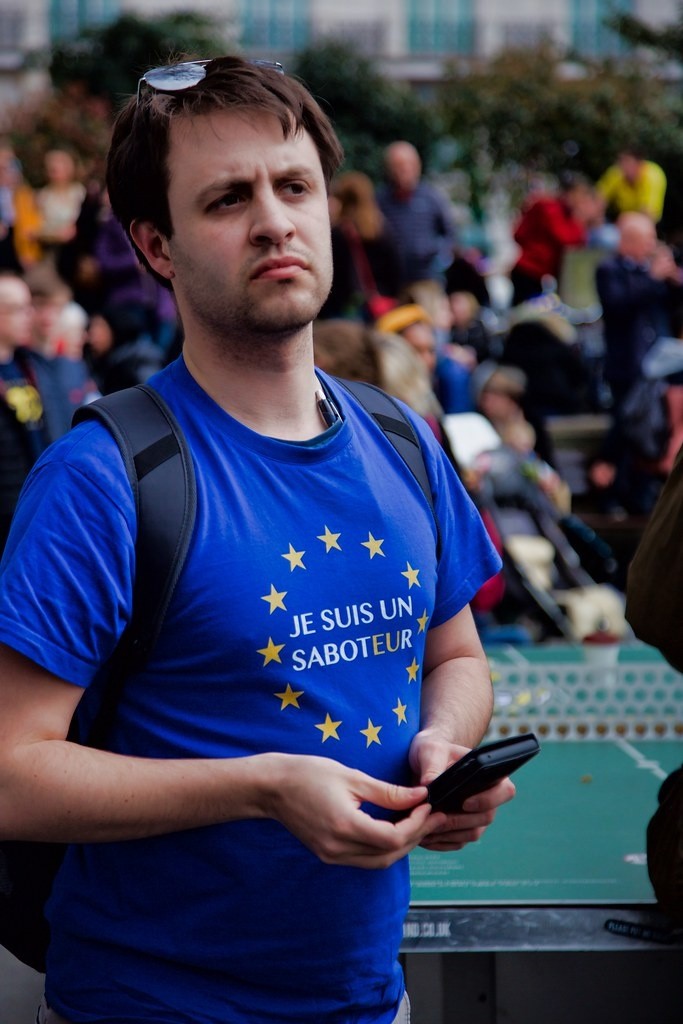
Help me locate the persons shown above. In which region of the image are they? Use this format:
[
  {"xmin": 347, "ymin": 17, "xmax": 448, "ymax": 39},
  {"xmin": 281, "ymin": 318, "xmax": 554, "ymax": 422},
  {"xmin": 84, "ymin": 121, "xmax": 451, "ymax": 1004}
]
[
  {"xmin": 332, "ymin": 143, "xmax": 683, "ymax": 417},
  {"xmin": 0, "ymin": 134, "xmax": 181, "ymax": 555},
  {"xmin": 0, "ymin": 42, "xmax": 517, "ymax": 1024},
  {"xmin": 432, "ymin": 389, "xmax": 683, "ymax": 1024}
]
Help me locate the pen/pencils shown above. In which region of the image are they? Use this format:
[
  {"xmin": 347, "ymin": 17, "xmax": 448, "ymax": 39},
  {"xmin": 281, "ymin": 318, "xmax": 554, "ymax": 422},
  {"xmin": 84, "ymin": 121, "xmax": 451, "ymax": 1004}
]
[{"xmin": 315, "ymin": 390, "xmax": 343, "ymax": 428}]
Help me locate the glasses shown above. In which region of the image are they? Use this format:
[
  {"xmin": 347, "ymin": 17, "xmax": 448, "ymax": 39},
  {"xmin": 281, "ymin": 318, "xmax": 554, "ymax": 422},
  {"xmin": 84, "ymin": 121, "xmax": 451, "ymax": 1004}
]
[{"xmin": 134, "ymin": 59, "xmax": 286, "ymax": 119}]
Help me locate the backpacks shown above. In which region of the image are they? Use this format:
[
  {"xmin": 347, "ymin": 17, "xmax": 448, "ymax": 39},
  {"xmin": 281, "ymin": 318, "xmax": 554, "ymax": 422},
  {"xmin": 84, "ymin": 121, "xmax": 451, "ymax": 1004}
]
[{"xmin": 1, "ymin": 382, "xmax": 435, "ymax": 975}]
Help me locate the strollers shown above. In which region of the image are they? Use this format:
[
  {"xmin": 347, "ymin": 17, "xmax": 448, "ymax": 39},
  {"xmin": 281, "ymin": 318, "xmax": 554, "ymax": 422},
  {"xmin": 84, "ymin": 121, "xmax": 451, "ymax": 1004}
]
[{"xmin": 469, "ymin": 468, "xmax": 637, "ymax": 644}]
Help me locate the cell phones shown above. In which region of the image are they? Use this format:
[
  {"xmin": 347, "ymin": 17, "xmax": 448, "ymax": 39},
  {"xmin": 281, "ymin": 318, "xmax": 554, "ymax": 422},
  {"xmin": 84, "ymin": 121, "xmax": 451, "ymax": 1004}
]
[{"xmin": 425, "ymin": 732, "xmax": 539, "ymax": 814}]
